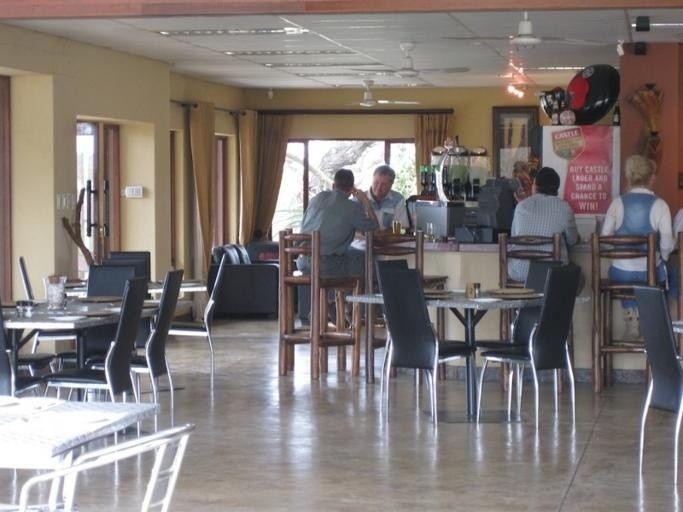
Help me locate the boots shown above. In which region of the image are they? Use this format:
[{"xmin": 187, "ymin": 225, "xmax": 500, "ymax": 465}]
[{"xmin": 622, "ymin": 307, "xmax": 640, "ymax": 341}]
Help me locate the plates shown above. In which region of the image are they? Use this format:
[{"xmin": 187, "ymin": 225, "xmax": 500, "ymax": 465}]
[
  {"xmin": 79, "ymin": 295, "xmax": 158, "ymax": 317},
  {"xmin": 424, "ymin": 288, "xmax": 545, "ymax": 300}
]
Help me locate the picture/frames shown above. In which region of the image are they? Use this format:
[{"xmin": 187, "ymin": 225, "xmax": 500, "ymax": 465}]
[{"xmin": 491, "ymin": 105, "xmax": 540, "ymax": 183}]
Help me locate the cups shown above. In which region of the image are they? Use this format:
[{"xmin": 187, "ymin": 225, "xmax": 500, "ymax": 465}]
[
  {"xmin": 392, "ymin": 219, "xmax": 401, "ymax": 235},
  {"xmin": 427, "ymin": 222, "xmax": 434, "ymax": 235},
  {"xmin": 43, "ymin": 276, "xmax": 68, "ymax": 310}
]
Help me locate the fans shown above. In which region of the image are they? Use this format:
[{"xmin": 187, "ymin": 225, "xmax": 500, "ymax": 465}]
[
  {"xmin": 439, "ymin": 11, "xmax": 621, "ymax": 48},
  {"xmin": 350, "ymin": 41, "xmax": 471, "ymax": 88},
  {"xmin": 338, "ymin": 79, "xmax": 421, "ymax": 108}
]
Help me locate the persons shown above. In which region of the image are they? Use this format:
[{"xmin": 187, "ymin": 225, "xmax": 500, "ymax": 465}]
[
  {"xmin": 599, "ymin": 154, "xmax": 677, "ymax": 343},
  {"xmin": 507, "ymin": 167, "xmax": 581, "ymax": 283},
  {"xmin": 296, "ymin": 168, "xmax": 379, "ymax": 329},
  {"xmin": 350, "ymin": 166, "xmax": 410, "ymax": 251}
]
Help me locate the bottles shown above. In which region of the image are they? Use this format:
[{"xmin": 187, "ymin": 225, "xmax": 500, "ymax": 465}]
[
  {"xmin": 474, "ymin": 283, "xmax": 481, "ymax": 297},
  {"xmin": 613, "ymin": 102, "xmax": 620, "ymax": 125},
  {"xmin": 15, "ymin": 300, "xmax": 24, "ymax": 312},
  {"xmin": 63, "ymin": 293, "xmax": 67, "ymax": 309},
  {"xmin": 420, "ymin": 165, "xmax": 429, "ymax": 193},
  {"xmin": 25, "ymin": 301, "xmax": 33, "ymax": 312}
]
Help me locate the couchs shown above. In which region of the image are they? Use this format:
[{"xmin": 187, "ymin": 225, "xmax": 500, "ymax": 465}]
[{"xmin": 207, "ymin": 241, "xmax": 279, "ymax": 320}]
